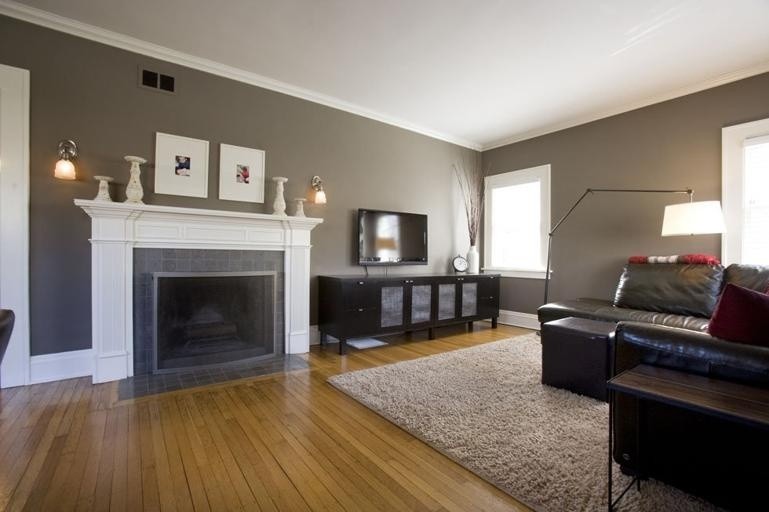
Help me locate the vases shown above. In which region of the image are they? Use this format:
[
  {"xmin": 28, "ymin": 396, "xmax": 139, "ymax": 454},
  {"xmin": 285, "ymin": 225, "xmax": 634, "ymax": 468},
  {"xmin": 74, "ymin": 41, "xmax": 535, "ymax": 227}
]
[{"xmin": 466, "ymin": 246, "xmax": 480, "ymax": 274}]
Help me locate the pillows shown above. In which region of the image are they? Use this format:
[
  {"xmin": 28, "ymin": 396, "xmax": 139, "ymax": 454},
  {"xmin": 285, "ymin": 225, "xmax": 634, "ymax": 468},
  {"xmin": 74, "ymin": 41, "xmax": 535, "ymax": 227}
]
[{"xmin": 707, "ymin": 282, "xmax": 768, "ymax": 347}]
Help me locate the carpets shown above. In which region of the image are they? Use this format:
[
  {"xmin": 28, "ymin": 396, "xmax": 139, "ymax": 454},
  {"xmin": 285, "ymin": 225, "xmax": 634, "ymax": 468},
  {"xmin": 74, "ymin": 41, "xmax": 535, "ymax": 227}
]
[{"xmin": 325, "ymin": 330, "xmax": 750, "ymax": 512}]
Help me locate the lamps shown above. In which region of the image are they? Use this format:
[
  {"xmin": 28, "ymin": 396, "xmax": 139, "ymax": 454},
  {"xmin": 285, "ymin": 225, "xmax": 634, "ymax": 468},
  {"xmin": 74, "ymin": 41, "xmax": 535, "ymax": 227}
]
[
  {"xmin": 52, "ymin": 139, "xmax": 80, "ymax": 181},
  {"xmin": 536, "ymin": 186, "xmax": 722, "ymax": 336},
  {"xmin": 310, "ymin": 175, "xmax": 327, "ymax": 205}
]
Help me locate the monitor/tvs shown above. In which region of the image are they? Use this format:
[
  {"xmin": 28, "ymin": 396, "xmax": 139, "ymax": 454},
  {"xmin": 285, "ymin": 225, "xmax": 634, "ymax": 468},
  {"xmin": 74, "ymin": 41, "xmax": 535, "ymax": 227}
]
[{"xmin": 357, "ymin": 207, "xmax": 428, "ymax": 266}]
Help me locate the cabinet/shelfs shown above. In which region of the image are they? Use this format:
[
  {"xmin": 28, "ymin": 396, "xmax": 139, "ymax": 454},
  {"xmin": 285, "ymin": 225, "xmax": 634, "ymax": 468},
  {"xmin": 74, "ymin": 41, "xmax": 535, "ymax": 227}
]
[
  {"xmin": 379, "ymin": 275, "xmax": 436, "ymax": 337},
  {"xmin": 318, "ymin": 275, "xmax": 379, "ymax": 356},
  {"xmin": 480, "ymin": 274, "xmax": 500, "ymax": 328},
  {"xmin": 436, "ymin": 276, "xmax": 479, "ymax": 327}
]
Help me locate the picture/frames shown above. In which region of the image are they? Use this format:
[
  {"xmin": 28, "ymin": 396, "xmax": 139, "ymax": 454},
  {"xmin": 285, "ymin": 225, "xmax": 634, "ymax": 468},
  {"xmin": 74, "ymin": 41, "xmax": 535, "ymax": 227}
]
[
  {"xmin": 217, "ymin": 142, "xmax": 266, "ymax": 203},
  {"xmin": 153, "ymin": 130, "xmax": 210, "ymax": 198}
]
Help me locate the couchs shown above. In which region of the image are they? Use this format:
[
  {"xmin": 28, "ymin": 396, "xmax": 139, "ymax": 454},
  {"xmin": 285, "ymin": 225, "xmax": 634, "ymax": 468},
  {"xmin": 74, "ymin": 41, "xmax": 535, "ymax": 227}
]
[
  {"xmin": 536, "ymin": 259, "xmax": 768, "ymax": 401},
  {"xmin": 540, "ymin": 316, "xmax": 616, "ymax": 404}
]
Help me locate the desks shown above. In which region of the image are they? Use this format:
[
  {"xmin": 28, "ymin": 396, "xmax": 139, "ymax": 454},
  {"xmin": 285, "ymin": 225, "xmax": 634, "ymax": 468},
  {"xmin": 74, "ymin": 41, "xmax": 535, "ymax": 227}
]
[{"xmin": 605, "ymin": 362, "xmax": 768, "ymax": 512}]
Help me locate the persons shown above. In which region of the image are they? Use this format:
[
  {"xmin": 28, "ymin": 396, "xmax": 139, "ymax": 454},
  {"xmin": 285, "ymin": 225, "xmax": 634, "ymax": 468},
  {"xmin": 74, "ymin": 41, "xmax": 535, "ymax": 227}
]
[
  {"xmin": 177, "ymin": 156, "xmax": 190, "ymax": 176},
  {"xmin": 240, "ymin": 167, "xmax": 249, "ymax": 183}
]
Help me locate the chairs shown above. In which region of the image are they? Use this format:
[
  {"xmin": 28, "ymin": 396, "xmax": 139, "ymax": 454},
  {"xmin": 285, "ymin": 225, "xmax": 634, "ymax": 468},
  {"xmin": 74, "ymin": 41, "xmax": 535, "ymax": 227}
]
[{"xmin": 0, "ymin": 308, "xmax": 15, "ymax": 364}]
